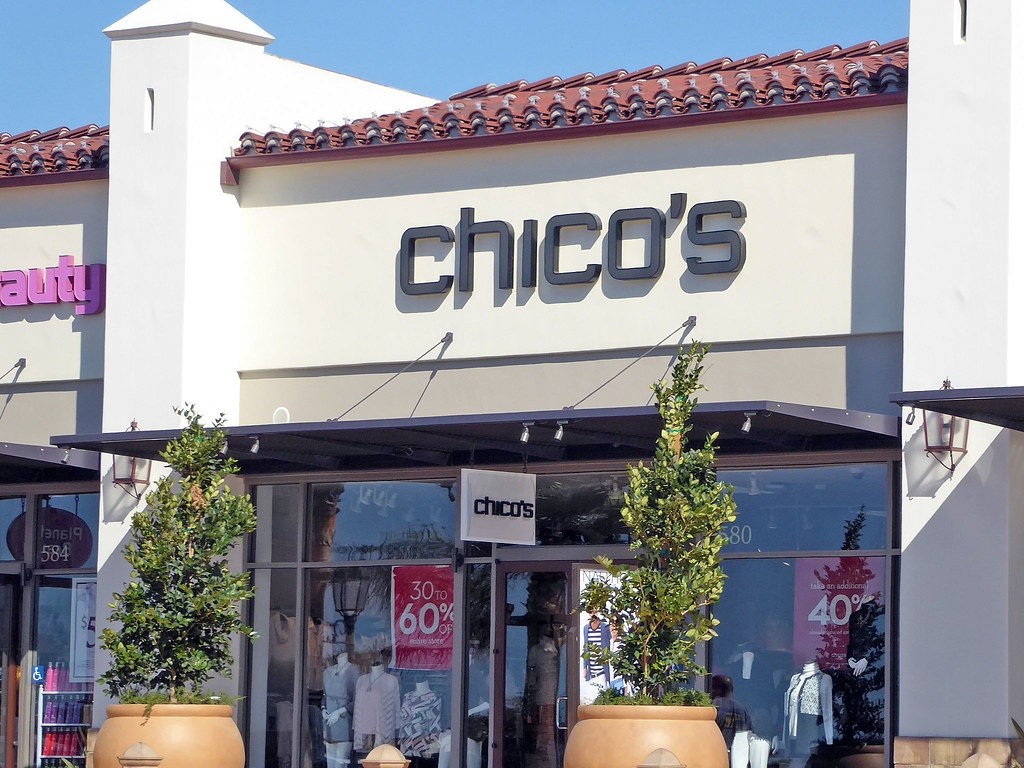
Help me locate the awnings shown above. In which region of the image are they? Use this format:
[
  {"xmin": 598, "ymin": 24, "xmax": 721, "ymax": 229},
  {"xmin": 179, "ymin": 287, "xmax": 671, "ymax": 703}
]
[{"xmin": 51, "ymin": 400, "xmax": 902, "ymax": 478}]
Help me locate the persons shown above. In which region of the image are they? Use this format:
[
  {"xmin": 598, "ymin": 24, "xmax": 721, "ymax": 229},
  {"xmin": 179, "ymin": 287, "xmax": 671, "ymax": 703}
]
[
  {"xmin": 842, "ymin": 594, "xmax": 887, "ymax": 746},
  {"xmin": 398, "ymin": 677, "xmax": 442, "ymax": 768},
  {"xmin": 781, "ymin": 660, "xmax": 834, "ymax": 767},
  {"xmin": 320, "ymin": 652, "xmax": 362, "ymax": 768},
  {"xmin": 727, "ymin": 652, "xmax": 781, "ymax": 767},
  {"xmin": 528, "ymin": 632, "xmax": 557, "ymax": 767},
  {"xmin": 709, "ymin": 675, "xmax": 753, "ymax": 750},
  {"xmin": 351, "ymin": 661, "xmax": 402, "ymax": 768},
  {"xmin": 582, "ymin": 619, "xmax": 612, "ymax": 702}
]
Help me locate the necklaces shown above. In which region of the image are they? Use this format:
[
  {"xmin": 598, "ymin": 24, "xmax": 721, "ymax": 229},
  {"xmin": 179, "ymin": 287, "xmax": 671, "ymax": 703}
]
[
  {"xmin": 366, "ymin": 671, "xmax": 386, "ymax": 692},
  {"xmin": 334, "ymin": 662, "xmax": 351, "ymax": 675}
]
